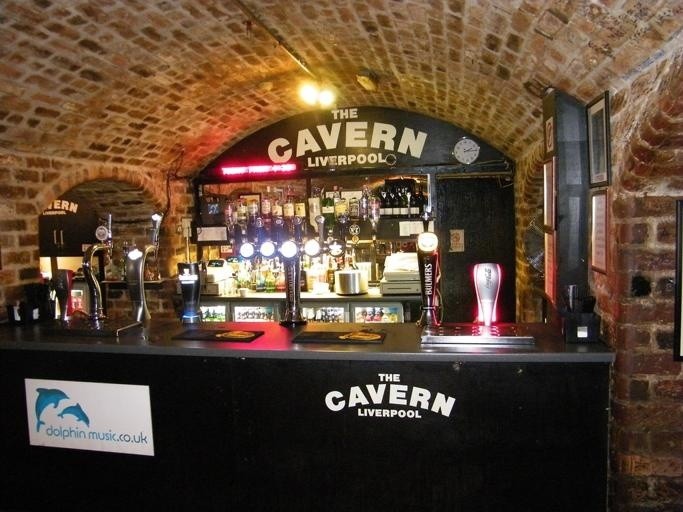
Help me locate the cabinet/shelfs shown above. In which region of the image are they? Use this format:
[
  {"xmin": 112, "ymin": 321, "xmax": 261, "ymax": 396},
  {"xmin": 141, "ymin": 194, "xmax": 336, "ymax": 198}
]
[{"xmin": 191, "ymin": 168, "xmax": 435, "ymax": 303}]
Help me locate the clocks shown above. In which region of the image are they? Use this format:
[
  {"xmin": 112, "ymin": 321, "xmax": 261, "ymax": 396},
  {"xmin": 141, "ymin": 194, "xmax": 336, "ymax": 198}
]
[{"xmin": 452, "ymin": 136, "xmax": 481, "ymax": 165}]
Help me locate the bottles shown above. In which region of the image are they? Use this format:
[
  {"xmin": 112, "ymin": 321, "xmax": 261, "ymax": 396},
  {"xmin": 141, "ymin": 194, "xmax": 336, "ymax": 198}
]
[
  {"xmin": 198, "ymin": 306, "xmax": 398, "ymax": 323},
  {"xmin": 224, "ymin": 183, "xmax": 360, "ymax": 292},
  {"xmin": 360, "ymin": 184, "xmax": 380, "ymax": 221},
  {"xmin": 381, "ymin": 179, "xmax": 427, "ymax": 219}
]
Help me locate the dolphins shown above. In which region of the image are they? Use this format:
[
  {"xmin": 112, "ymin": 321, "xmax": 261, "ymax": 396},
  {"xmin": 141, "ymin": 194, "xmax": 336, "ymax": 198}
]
[
  {"xmin": 56, "ymin": 402, "xmax": 90, "ymax": 429},
  {"xmin": 33, "ymin": 387, "xmax": 70, "ymax": 433}
]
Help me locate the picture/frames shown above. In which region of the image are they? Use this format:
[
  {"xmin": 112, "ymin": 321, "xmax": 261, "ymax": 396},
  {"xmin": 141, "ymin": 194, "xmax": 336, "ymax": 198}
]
[
  {"xmin": 543, "ymin": 228, "xmax": 557, "ymax": 310},
  {"xmin": 542, "ymin": 155, "xmax": 558, "ymax": 231},
  {"xmin": 585, "ymin": 90, "xmax": 613, "ymax": 188},
  {"xmin": 591, "ymin": 189, "xmax": 609, "ymax": 276}
]
